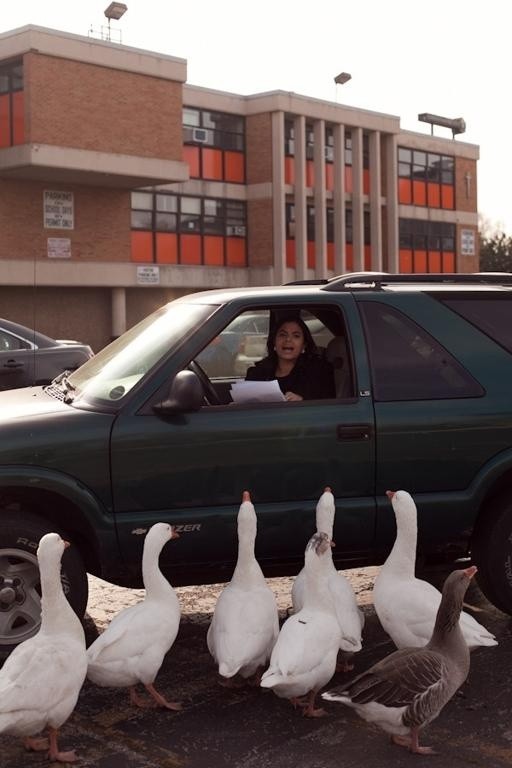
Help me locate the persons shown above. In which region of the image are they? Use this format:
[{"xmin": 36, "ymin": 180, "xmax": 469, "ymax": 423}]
[{"xmin": 244, "ymin": 318, "xmax": 336, "ymax": 399}]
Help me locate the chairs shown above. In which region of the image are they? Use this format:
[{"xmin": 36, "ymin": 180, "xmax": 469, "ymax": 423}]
[{"xmin": 326, "ymin": 337, "xmax": 352, "ymax": 398}]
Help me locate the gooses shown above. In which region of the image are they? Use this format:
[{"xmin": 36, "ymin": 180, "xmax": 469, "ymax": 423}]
[
  {"xmin": 370, "ymin": 488, "xmax": 499, "ymax": 702},
  {"xmin": 0, "ymin": 532, "xmax": 87, "ymax": 764},
  {"xmin": 320, "ymin": 565, "xmax": 479, "ymax": 756},
  {"xmin": 205, "ymin": 488, "xmax": 283, "ymax": 691},
  {"xmin": 290, "ymin": 484, "xmax": 367, "ymax": 675},
  {"xmin": 85, "ymin": 520, "xmax": 187, "ymax": 713},
  {"xmin": 257, "ymin": 532, "xmax": 341, "ymax": 722}
]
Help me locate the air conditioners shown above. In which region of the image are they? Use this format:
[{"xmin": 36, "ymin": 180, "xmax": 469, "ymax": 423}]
[
  {"xmin": 185, "ymin": 127, "xmax": 208, "ymax": 144},
  {"xmin": 233, "ymin": 226, "xmax": 246, "ymax": 236}
]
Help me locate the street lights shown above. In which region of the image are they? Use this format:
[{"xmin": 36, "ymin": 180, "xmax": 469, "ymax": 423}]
[
  {"xmin": 334, "ymin": 72, "xmax": 352, "ymax": 102},
  {"xmin": 104, "ymin": 1, "xmax": 127, "ymax": 41}
]
